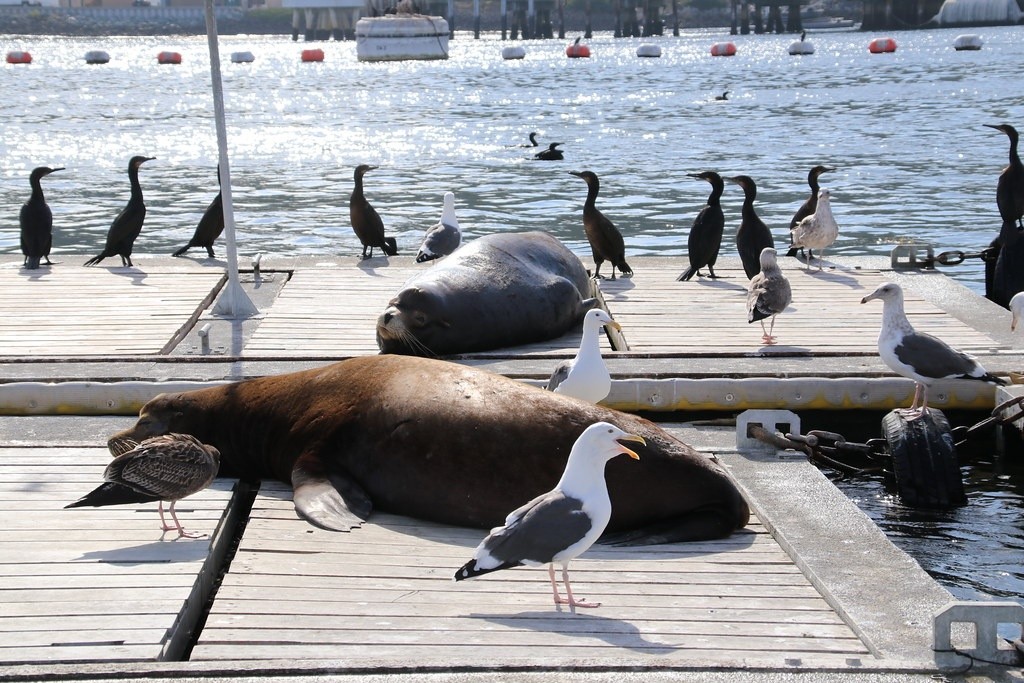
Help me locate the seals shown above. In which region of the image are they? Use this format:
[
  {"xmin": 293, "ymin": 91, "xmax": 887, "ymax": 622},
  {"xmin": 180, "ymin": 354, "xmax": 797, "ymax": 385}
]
[
  {"xmin": 375, "ymin": 230, "xmax": 599, "ymax": 355},
  {"xmin": 109, "ymin": 355, "xmax": 749, "ymax": 548}
]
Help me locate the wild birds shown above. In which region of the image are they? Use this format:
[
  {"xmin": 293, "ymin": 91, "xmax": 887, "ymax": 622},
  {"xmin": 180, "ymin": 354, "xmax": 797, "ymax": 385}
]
[
  {"xmin": 64, "ymin": 428, "xmax": 224, "ymax": 538},
  {"xmin": 982, "ymin": 122, "xmax": 1024, "ymax": 231},
  {"xmin": 18, "ymin": 153, "xmax": 225, "ymax": 268},
  {"xmin": 454, "ymin": 422, "xmax": 647, "ymax": 607},
  {"xmin": 861, "ymin": 279, "xmax": 1008, "ymax": 422},
  {"xmin": 546, "ymin": 308, "xmax": 623, "ymax": 405},
  {"xmin": 352, "ymin": 130, "xmax": 841, "ymax": 344}
]
